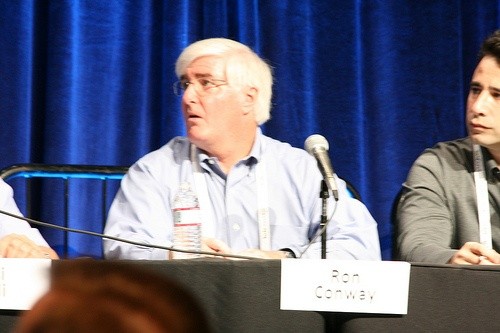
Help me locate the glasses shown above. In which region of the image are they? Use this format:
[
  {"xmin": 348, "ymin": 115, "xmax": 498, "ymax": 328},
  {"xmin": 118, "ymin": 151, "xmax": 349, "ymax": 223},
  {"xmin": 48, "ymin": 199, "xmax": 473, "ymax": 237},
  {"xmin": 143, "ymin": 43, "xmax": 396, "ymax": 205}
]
[{"xmin": 172, "ymin": 76, "xmax": 227, "ymax": 97}]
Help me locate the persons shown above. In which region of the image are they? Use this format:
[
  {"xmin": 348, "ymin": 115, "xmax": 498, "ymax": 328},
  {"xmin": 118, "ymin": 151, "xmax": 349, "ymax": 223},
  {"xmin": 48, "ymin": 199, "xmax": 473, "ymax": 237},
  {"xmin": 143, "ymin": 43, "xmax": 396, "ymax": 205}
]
[
  {"xmin": 391, "ymin": 32, "xmax": 500, "ymax": 271},
  {"xmin": 0, "ymin": 170, "xmax": 63, "ymax": 263},
  {"xmin": 94, "ymin": 33, "xmax": 389, "ymax": 269}
]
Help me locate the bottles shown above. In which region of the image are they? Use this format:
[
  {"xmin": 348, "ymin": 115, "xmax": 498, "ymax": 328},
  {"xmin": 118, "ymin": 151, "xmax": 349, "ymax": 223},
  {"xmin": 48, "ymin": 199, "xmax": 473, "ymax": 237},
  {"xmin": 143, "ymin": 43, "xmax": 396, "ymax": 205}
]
[{"xmin": 171, "ymin": 182, "xmax": 201, "ymax": 259}]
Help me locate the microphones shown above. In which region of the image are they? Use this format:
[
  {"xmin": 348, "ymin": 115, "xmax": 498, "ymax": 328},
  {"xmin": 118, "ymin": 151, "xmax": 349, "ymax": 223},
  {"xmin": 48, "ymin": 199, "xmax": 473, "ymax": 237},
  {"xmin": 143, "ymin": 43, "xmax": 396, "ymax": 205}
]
[{"xmin": 304, "ymin": 134, "xmax": 339, "ymax": 202}]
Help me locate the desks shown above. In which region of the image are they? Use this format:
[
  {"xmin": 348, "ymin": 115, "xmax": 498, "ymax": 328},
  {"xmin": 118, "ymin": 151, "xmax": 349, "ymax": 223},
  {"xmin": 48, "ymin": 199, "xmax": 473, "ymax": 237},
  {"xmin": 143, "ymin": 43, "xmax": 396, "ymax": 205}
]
[{"xmin": 1, "ymin": 259, "xmax": 499, "ymax": 333}]
